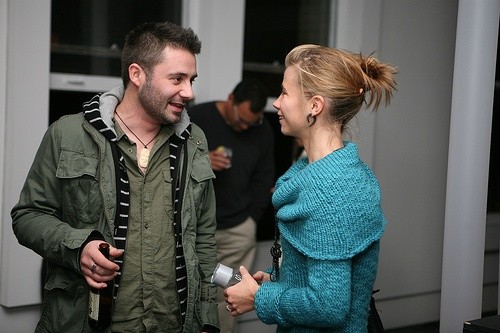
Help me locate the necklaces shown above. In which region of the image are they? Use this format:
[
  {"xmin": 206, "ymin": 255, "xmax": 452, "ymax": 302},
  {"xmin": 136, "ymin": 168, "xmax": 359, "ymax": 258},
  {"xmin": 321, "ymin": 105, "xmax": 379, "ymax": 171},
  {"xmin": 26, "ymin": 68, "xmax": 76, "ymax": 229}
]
[{"xmin": 115, "ymin": 105, "xmax": 159, "ymax": 167}]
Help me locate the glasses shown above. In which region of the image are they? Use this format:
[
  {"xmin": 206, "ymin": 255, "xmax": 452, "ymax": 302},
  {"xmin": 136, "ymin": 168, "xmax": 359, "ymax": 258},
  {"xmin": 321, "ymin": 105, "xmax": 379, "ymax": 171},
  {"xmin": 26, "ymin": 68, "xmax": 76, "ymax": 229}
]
[{"xmin": 232, "ymin": 105, "xmax": 263, "ymax": 128}]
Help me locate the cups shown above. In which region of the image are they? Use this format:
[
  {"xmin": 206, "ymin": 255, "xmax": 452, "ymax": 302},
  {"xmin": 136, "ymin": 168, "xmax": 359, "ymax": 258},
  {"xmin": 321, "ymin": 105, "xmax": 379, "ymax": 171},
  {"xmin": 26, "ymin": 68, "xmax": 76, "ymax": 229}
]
[{"xmin": 211, "ymin": 263, "xmax": 263, "ymax": 293}]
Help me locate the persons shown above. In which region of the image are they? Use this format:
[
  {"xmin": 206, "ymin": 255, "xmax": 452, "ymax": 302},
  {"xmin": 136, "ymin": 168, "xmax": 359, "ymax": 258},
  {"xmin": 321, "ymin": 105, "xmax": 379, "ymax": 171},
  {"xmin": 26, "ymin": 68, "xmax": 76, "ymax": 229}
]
[
  {"xmin": 12, "ymin": 23, "xmax": 219, "ymax": 333},
  {"xmin": 223, "ymin": 44, "xmax": 398, "ymax": 333},
  {"xmin": 181, "ymin": 79, "xmax": 268, "ymax": 333}
]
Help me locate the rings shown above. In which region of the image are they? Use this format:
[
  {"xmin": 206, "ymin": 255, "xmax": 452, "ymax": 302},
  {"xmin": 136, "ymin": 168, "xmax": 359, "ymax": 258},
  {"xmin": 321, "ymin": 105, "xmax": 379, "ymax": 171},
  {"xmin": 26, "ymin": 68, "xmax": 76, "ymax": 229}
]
[
  {"xmin": 89, "ymin": 259, "xmax": 100, "ymax": 274},
  {"xmin": 226, "ymin": 305, "xmax": 231, "ymax": 311}
]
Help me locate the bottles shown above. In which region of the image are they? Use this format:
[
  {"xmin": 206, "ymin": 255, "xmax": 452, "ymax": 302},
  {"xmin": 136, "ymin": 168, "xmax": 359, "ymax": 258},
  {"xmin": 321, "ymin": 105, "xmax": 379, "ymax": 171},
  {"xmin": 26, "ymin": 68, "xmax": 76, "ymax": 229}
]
[{"xmin": 86, "ymin": 244, "xmax": 114, "ymax": 333}]
